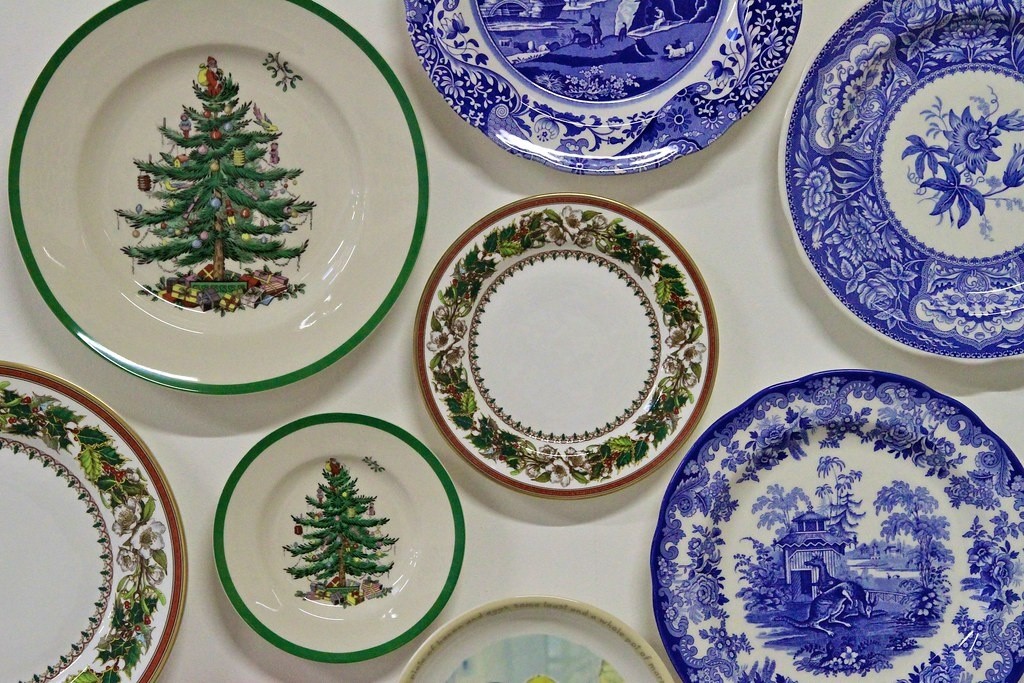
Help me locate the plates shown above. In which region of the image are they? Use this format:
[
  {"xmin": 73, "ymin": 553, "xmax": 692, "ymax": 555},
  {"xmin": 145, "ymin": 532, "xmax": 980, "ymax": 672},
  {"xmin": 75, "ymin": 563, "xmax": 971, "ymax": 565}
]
[
  {"xmin": 9, "ymin": 0, "xmax": 429, "ymax": 396},
  {"xmin": 413, "ymin": 192, "xmax": 719, "ymax": 498},
  {"xmin": 401, "ymin": 597, "xmax": 674, "ymax": 683},
  {"xmin": 650, "ymin": 368, "xmax": 1024, "ymax": 683},
  {"xmin": 0, "ymin": 361, "xmax": 188, "ymax": 683},
  {"xmin": 403, "ymin": 0, "xmax": 804, "ymax": 180},
  {"xmin": 778, "ymin": 0, "xmax": 1024, "ymax": 362},
  {"xmin": 214, "ymin": 412, "xmax": 467, "ymax": 663}
]
